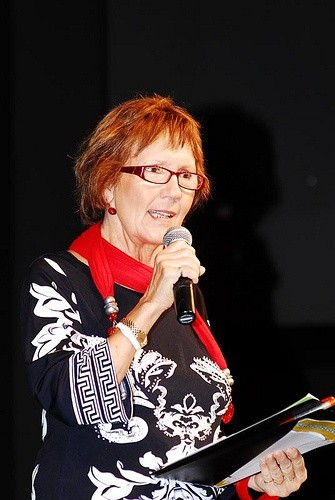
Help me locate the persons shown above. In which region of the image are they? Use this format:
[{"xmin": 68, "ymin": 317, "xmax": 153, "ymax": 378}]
[{"xmin": 23, "ymin": 94, "xmax": 310, "ymax": 500}]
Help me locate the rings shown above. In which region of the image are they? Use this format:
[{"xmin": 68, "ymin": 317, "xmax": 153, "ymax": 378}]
[
  {"xmin": 263, "ymin": 478, "xmax": 275, "ymax": 485},
  {"xmin": 177, "ymin": 265, "xmax": 184, "ymax": 278},
  {"xmin": 283, "ymin": 472, "xmax": 296, "ymax": 481},
  {"xmin": 273, "ymin": 479, "xmax": 286, "ymax": 486}
]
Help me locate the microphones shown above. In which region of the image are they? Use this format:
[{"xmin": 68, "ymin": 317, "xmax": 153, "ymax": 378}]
[{"xmin": 163, "ymin": 225, "xmax": 196, "ymax": 325}]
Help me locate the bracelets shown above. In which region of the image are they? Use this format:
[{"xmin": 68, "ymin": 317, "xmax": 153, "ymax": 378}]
[
  {"xmin": 115, "ymin": 322, "xmax": 143, "ymax": 351},
  {"xmin": 120, "ymin": 318, "xmax": 149, "ymax": 348}
]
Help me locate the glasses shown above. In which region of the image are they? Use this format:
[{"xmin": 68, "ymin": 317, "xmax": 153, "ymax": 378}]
[{"xmin": 120, "ymin": 165, "xmax": 205, "ymax": 191}]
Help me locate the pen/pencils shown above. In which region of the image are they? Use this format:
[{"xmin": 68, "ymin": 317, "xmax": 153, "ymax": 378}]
[{"xmin": 278, "ymin": 396, "xmax": 335, "ymax": 426}]
